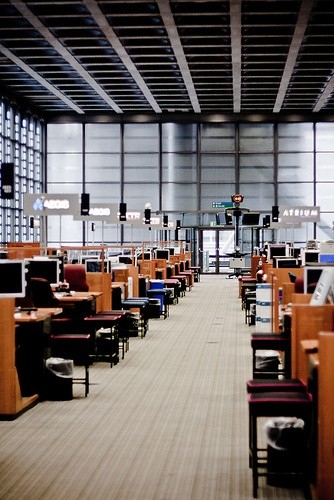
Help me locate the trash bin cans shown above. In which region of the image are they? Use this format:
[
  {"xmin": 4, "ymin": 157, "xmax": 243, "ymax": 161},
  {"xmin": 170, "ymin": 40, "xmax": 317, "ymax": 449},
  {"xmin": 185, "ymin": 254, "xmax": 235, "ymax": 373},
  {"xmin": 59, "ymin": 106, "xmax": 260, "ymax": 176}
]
[
  {"xmin": 43, "ymin": 358, "xmax": 73, "ymax": 400},
  {"xmin": 128, "ymin": 312, "xmax": 139, "ymax": 337},
  {"xmin": 96, "ymin": 328, "xmax": 114, "ymax": 355},
  {"xmin": 250, "ymin": 304, "xmax": 256, "ymax": 325},
  {"xmin": 149, "ymin": 299, "xmax": 161, "ymax": 318},
  {"xmin": 163, "ymin": 288, "xmax": 174, "ymax": 305},
  {"xmin": 256, "ymin": 353, "xmax": 279, "ymax": 380},
  {"xmin": 149, "ymin": 279, "xmax": 164, "ymax": 311},
  {"xmin": 194, "ymin": 273, "xmax": 198, "ymax": 282},
  {"xmin": 246, "ymin": 292, "xmax": 256, "ymax": 297},
  {"xmin": 267, "ymin": 417, "xmax": 305, "ymax": 489},
  {"xmin": 177, "ymin": 282, "xmax": 181, "ymax": 297}
]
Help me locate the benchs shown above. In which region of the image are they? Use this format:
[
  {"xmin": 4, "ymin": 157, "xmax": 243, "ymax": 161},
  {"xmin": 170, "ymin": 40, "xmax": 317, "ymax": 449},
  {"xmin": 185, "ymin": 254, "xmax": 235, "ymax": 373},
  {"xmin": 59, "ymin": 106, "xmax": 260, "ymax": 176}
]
[{"xmin": 51, "ymin": 266, "xmax": 202, "ymax": 398}]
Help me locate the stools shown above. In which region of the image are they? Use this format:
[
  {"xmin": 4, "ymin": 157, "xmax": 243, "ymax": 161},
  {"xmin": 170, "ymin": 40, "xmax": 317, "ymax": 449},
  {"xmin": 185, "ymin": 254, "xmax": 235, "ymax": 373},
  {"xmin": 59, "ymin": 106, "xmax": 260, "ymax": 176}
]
[
  {"xmin": 239, "ymin": 276, "xmax": 318, "ymax": 499},
  {"xmin": 14, "ymin": 258, "xmax": 195, "ymax": 323}
]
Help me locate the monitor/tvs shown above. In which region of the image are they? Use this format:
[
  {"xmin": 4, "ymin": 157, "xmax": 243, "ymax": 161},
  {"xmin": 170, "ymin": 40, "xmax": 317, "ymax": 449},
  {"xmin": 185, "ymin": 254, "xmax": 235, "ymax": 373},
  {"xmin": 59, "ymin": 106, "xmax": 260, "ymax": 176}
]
[
  {"xmin": 265, "ymin": 243, "xmax": 334, "ymax": 292},
  {"xmin": 0, "ymin": 247, "xmax": 175, "ymax": 298},
  {"xmin": 241, "ymin": 212, "xmax": 260, "ymax": 226}
]
